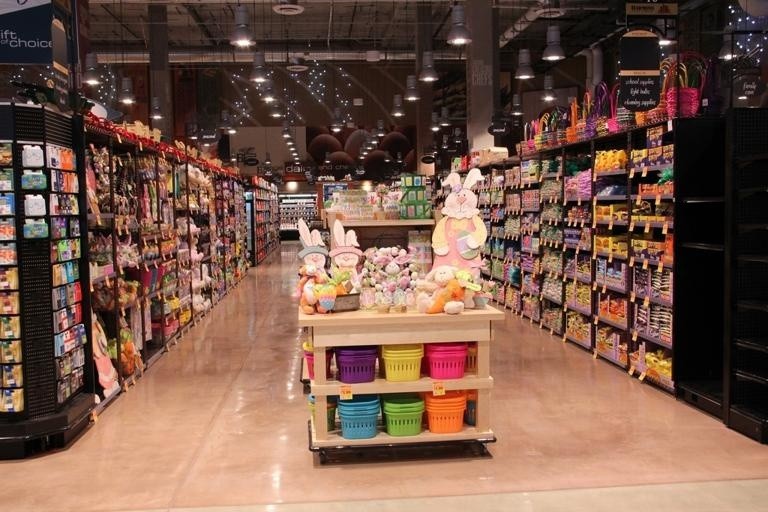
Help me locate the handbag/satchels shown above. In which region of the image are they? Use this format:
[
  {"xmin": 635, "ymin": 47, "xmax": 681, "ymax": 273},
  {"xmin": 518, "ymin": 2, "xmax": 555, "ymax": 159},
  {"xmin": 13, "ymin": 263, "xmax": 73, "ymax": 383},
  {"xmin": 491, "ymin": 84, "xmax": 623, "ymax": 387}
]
[{"xmin": 516, "ymin": 53, "xmax": 708, "ymax": 158}]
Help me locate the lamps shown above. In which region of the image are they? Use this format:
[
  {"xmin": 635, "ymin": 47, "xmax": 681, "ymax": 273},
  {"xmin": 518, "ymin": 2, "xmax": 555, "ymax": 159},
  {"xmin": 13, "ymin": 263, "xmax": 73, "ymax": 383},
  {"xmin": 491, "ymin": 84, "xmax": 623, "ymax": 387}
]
[
  {"xmin": 358, "ymin": 120, "xmax": 385, "ymax": 159},
  {"xmin": 118, "ymin": 77, "xmax": 135, "ymax": 105},
  {"xmin": 403, "ymin": 75, "xmax": 421, "ymax": 101},
  {"xmin": 81, "ymin": 53, "xmax": 100, "ymax": 84},
  {"xmin": 148, "ymin": 97, "xmax": 166, "ymax": 119},
  {"xmin": 541, "ymin": 25, "xmax": 567, "ymax": 61},
  {"xmin": 390, "ymin": 94, "xmax": 406, "ymax": 117},
  {"xmin": 250, "ymin": 51, "xmax": 269, "ymax": 84},
  {"xmin": 539, "ymin": 75, "xmax": 559, "ymax": 102},
  {"xmin": 430, "ymin": 112, "xmax": 442, "ymax": 132},
  {"xmin": 384, "ymin": 151, "xmax": 392, "ymax": 163},
  {"xmin": 262, "ymin": 86, "xmax": 283, "ymax": 120},
  {"xmin": 439, "ymin": 106, "xmax": 453, "ymax": 126},
  {"xmin": 282, "ymin": 120, "xmax": 301, "ymax": 166},
  {"xmin": 446, "ymin": 5, "xmax": 473, "ymax": 45},
  {"xmin": 230, "ymin": 6, "xmax": 257, "ymax": 48},
  {"xmin": 717, "ymin": 26, "xmax": 744, "ymax": 61},
  {"xmin": 396, "ymin": 152, "xmax": 404, "ymax": 163},
  {"xmin": 325, "ymin": 152, "xmax": 331, "ymax": 164},
  {"xmin": 655, "ymin": 18, "xmax": 676, "ymax": 46},
  {"xmin": 515, "ymin": 48, "xmax": 536, "ymax": 79},
  {"xmin": 510, "ymin": 94, "xmax": 525, "ymax": 116}
]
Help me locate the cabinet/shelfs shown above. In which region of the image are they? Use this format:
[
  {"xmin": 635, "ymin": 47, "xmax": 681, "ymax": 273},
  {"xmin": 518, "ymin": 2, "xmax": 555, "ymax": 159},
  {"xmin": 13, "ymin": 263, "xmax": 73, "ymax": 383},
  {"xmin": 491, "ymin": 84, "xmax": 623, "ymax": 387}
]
[
  {"xmin": 278, "ymin": 192, "xmax": 321, "ymax": 241},
  {"xmin": 298, "ymin": 304, "xmax": 505, "ymax": 465},
  {"xmin": 247, "ymin": 175, "xmax": 280, "ymax": 267}
]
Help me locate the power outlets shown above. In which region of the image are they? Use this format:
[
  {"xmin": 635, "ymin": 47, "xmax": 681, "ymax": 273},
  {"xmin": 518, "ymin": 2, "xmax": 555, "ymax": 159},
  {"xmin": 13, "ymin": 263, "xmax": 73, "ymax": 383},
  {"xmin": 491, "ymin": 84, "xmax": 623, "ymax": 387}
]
[{"xmin": 418, "ymin": 51, "xmax": 439, "ymax": 82}]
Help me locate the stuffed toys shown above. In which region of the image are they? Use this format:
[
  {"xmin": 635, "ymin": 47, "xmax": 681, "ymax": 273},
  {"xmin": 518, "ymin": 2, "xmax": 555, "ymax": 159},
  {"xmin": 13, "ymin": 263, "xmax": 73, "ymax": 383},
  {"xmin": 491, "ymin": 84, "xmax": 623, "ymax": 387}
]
[
  {"xmin": 299, "ymin": 261, "xmax": 335, "ymax": 314},
  {"xmin": 360, "ymin": 245, "xmax": 415, "ymax": 307},
  {"xmin": 295, "ymin": 218, "xmax": 330, "ymax": 276},
  {"xmin": 329, "ymin": 218, "xmax": 362, "ymax": 295},
  {"xmin": 415, "ymin": 265, "xmax": 465, "ymax": 314},
  {"xmin": 432, "ymin": 168, "xmax": 487, "ymax": 282}
]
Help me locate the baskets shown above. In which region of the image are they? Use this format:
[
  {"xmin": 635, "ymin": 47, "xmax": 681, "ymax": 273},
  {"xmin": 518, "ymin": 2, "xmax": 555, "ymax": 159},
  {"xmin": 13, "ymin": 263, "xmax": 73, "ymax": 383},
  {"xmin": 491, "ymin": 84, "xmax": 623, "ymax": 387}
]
[{"xmin": 305, "ymin": 344, "xmax": 479, "ymax": 440}]
